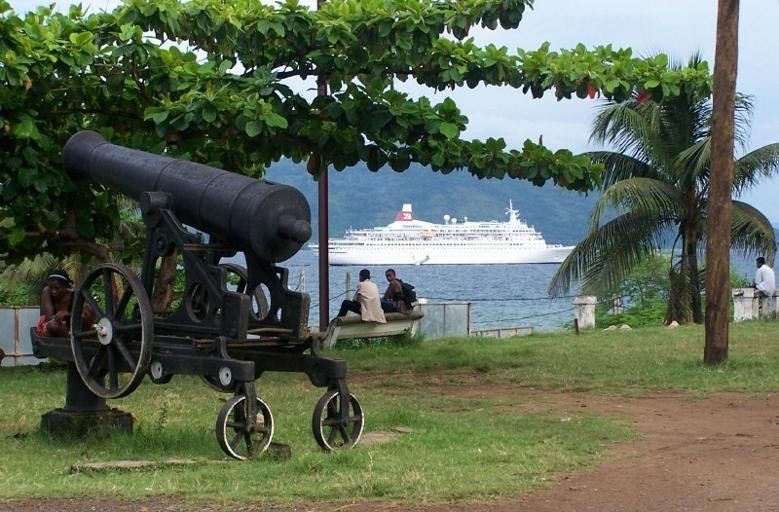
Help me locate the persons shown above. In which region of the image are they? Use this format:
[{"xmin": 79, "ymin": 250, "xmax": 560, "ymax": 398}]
[
  {"xmin": 338, "ymin": 269, "xmax": 388, "ymax": 324},
  {"xmin": 380, "ymin": 268, "xmax": 418, "ymax": 316},
  {"xmin": 39, "ymin": 269, "xmax": 95, "ymax": 337},
  {"xmin": 750, "ymin": 256, "xmax": 776, "ymax": 297}
]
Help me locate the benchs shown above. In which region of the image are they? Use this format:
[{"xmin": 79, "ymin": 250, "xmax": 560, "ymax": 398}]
[{"xmin": 310, "ymin": 311, "xmax": 424, "ymax": 350}]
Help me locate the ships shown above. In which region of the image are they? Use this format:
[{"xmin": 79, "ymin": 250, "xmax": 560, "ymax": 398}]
[{"xmin": 302, "ymin": 196, "xmax": 578, "ymax": 267}]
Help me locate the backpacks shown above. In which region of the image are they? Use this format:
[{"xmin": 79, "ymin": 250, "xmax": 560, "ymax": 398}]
[{"xmin": 391, "ymin": 278, "xmax": 419, "ymax": 310}]
